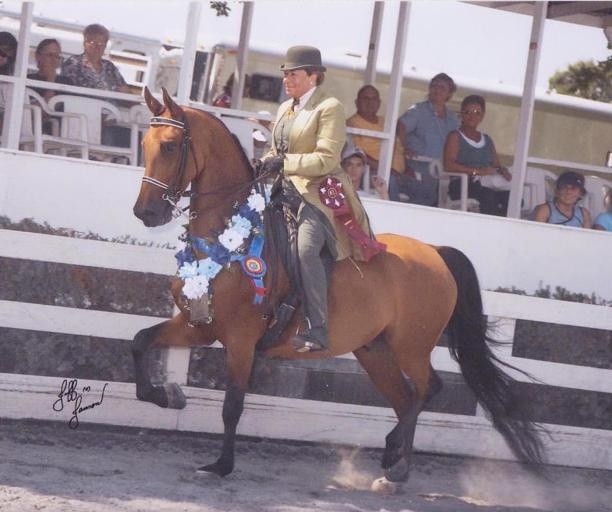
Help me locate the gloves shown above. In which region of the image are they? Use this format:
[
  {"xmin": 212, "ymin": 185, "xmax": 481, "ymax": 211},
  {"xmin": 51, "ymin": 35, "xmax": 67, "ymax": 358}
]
[{"xmin": 262, "ymin": 157, "xmax": 282, "ymax": 172}]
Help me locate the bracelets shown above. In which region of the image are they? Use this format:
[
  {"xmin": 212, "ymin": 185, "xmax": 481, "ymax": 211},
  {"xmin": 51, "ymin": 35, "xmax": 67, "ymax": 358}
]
[
  {"xmin": 497, "ymin": 165, "xmax": 507, "ymax": 172},
  {"xmin": 471, "ymin": 167, "xmax": 480, "ymax": 177}
]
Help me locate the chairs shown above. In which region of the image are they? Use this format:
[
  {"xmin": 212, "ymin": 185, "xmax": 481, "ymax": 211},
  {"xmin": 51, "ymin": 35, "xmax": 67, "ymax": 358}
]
[
  {"xmin": 221, "ymin": 118, "xmax": 275, "ymax": 161},
  {"xmin": 403, "ymin": 152, "xmax": 445, "ymax": 191},
  {"xmin": 522, "ymin": 165, "xmax": 562, "ymax": 214},
  {"xmin": 351, "ymin": 150, "xmax": 422, "ymax": 202},
  {"xmin": 583, "ymin": 174, "xmax": 612, "ymax": 223},
  {"xmin": 426, "ymin": 157, "xmax": 510, "ymax": 215},
  {"xmin": 45, "ymin": 94, "xmax": 141, "ymax": 167},
  {"xmin": 2, "ymin": 86, "xmax": 87, "ymax": 160}
]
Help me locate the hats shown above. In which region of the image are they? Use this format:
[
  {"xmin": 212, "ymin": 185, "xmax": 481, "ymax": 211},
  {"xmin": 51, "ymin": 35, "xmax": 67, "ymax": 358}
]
[
  {"xmin": 556, "ymin": 171, "xmax": 586, "ymax": 194},
  {"xmin": 279, "ymin": 45, "xmax": 327, "ymax": 72},
  {"xmin": 342, "ymin": 147, "xmax": 367, "ymax": 164},
  {"xmin": 248, "ymin": 110, "xmax": 273, "ymax": 122}
]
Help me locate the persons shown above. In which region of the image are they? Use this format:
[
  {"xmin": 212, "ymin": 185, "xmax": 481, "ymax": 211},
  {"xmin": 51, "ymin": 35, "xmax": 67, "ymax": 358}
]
[
  {"xmin": 1, "ymin": 32, "xmax": 20, "ymax": 136},
  {"xmin": 341, "ymin": 144, "xmax": 390, "ymax": 200},
  {"xmin": 443, "ymin": 93, "xmax": 525, "ymax": 218},
  {"xmin": 596, "ymin": 183, "xmax": 612, "ymax": 233},
  {"xmin": 527, "ymin": 170, "xmax": 595, "ymax": 231},
  {"xmin": 27, "ymin": 37, "xmax": 81, "ymax": 139},
  {"xmin": 211, "ymin": 71, "xmax": 258, "ymax": 125},
  {"xmin": 62, "ymin": 22, "xmax": 146, "ymax": 167},
  {"xmin": 396, "ymin": 72, "xmax": 462, "ymax": 181},
  {"xmin": 345, "ymin": 85, "xmax": 438, "ymax": 206},
  {"xmin": 248, "ymin": 109, "xmax": 274, "ymax": 161},
  {"xmin": 250, "ymin": 44, "xmax": 377, "ymax": 356}
]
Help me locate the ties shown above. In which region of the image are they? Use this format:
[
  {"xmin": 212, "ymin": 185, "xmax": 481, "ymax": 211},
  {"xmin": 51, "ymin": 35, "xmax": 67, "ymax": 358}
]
[{"xmin": 284, "ymin": 100, "xmax": 300, "ymax": 127}]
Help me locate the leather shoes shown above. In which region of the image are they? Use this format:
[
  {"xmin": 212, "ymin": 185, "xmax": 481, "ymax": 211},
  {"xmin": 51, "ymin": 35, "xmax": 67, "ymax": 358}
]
[{"xmin": 292, "ymin": 326, "xmax": 328, "ymax": 352}]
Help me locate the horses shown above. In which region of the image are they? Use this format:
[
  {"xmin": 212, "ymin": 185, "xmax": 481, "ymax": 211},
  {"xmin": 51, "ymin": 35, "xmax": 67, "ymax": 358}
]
[{"xmin": 130, "ymin": 85, "xmax": 557, "ymax": 496}]
